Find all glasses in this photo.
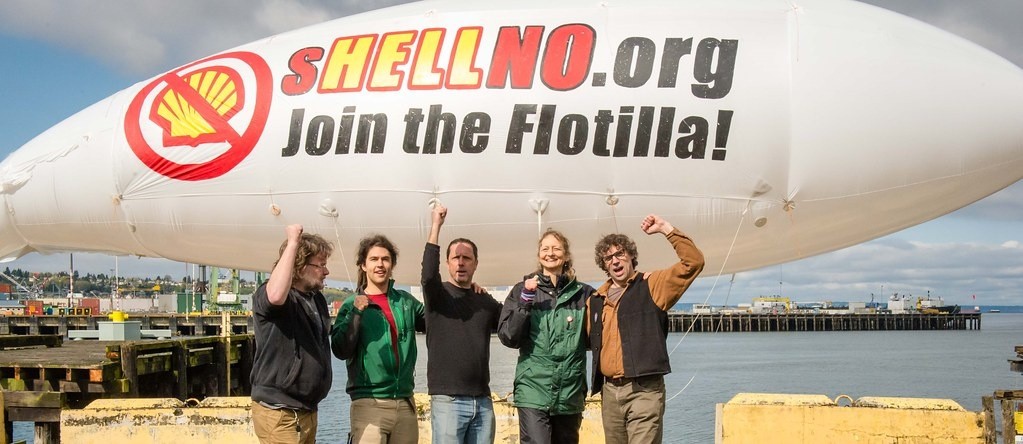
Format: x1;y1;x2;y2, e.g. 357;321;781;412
306;262;326;271
602;247;626;264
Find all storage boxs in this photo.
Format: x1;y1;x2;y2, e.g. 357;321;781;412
22;300;44;314
81;299;101;316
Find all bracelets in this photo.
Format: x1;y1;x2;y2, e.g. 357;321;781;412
521;293;535;301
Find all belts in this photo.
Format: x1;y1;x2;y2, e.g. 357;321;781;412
607;375;633;386
404;397;415;412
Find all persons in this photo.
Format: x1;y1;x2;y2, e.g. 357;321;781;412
331;234;426;444
249;224;334;444
585;214;705;444
421;206;504;444
497;230;597;444
